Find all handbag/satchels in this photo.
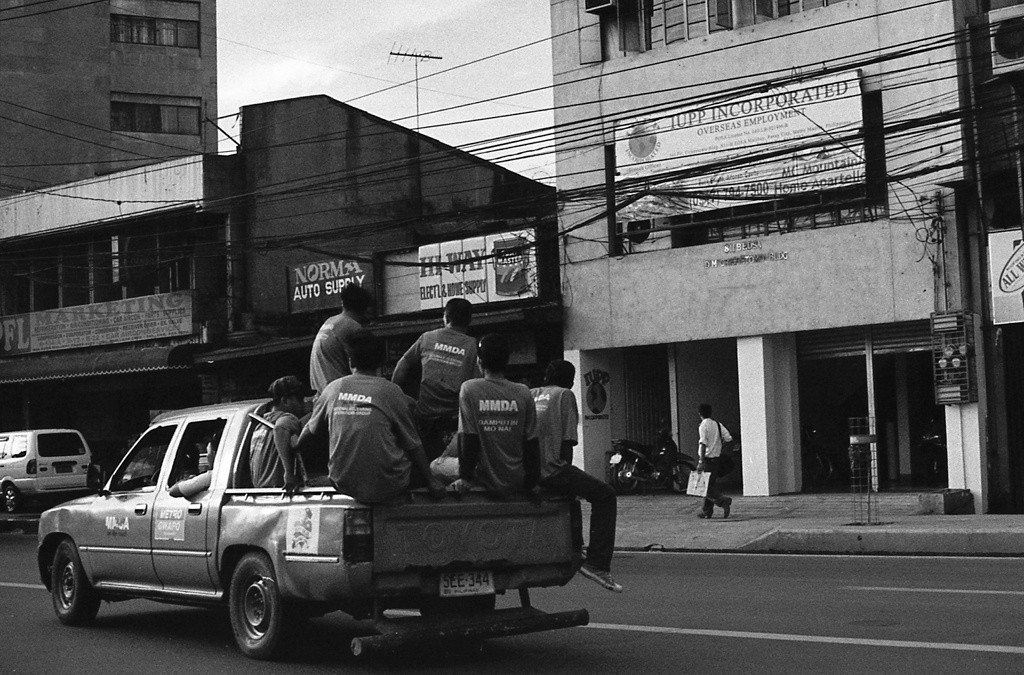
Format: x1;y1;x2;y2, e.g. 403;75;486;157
686;470;713;498
715;454;734;478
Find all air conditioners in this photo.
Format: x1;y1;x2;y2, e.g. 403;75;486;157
622;218;672;254
988;4;1024;75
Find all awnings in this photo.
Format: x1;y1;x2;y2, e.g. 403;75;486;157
0;343;207;385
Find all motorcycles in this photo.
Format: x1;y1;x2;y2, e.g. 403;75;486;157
607;420;696;495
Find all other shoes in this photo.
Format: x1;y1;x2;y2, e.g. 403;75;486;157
723;498;733;520
697;511;709;519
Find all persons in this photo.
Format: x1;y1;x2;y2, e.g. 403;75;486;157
693;402;733;518
290;328;447;507
428;332;625;594
249;375;308;497
169;438;218;499
393;296;480;437
310;282;370;394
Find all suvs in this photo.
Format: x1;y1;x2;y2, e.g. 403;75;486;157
0;430;101;514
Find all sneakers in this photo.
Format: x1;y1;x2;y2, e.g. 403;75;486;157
581;563;623;593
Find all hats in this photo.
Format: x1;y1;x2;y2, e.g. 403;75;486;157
269;377;317;400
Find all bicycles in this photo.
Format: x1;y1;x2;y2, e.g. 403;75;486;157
800;428;835;487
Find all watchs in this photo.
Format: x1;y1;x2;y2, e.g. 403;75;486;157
698;459;704;464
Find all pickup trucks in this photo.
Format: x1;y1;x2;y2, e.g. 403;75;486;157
34;396;591;658
